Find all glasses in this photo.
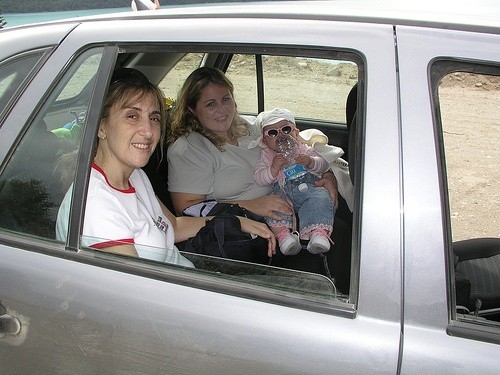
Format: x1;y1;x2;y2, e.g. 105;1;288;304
262;125;293;138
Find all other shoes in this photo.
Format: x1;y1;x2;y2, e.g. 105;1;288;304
307;229;335;255
279;230;302;255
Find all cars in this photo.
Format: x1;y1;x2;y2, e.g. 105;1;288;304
1;0;498;375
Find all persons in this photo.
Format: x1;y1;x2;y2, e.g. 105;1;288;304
165;66;339;257
54;67;278;269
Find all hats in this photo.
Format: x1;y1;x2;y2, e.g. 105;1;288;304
247;108;295;151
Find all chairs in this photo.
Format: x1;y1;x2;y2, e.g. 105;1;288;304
347;81;500;322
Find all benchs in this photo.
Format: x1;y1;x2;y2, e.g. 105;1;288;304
0;109;177;238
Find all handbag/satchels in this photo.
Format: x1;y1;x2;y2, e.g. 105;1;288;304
185;214;272;265
